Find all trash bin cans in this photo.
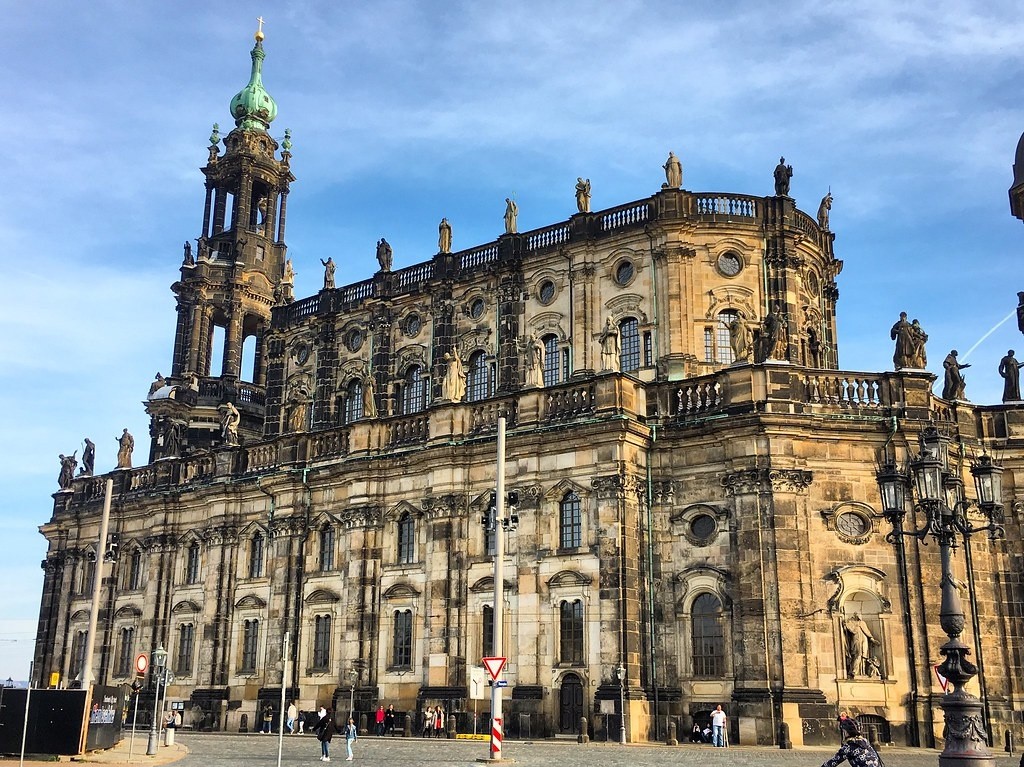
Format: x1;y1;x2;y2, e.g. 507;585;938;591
164;728;175;747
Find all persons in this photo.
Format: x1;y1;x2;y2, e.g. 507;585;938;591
840;610;882;676
148;372;180;457
165;710;181;731
821;718;886;767
438;218;452;254
57;437;96;490
260;700;357;761
773;155;834;231
286;345;466;432
575;177;591;212
891;312;1024;402
375;704;396;737
320;257;337;289
422;706;444;738
662;151;682;188
692;705;726;748
183;234;248;265
721;305;788;364
286;253;294;284
598;316;622;372
221;402;240;446
375;238;392;271
115;428;134;469
516;334;545;387
503;198;518;234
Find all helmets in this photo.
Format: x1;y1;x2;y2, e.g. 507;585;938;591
841;718;861;736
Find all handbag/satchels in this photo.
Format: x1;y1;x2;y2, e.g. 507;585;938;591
317;733;323;741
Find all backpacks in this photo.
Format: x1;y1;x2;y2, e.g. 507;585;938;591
267;708;273;717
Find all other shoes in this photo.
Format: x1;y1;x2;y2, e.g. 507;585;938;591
291;729;294;735
260;730;264;734
319;755;329;761
346;757;353;761
268;731;271;733
297;732;304;734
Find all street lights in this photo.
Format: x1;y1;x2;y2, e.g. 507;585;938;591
475;492;518;764
79;532;119;690
348;669;360;718
146;642;168;754
871;413;1005;767
616;661;627;743
